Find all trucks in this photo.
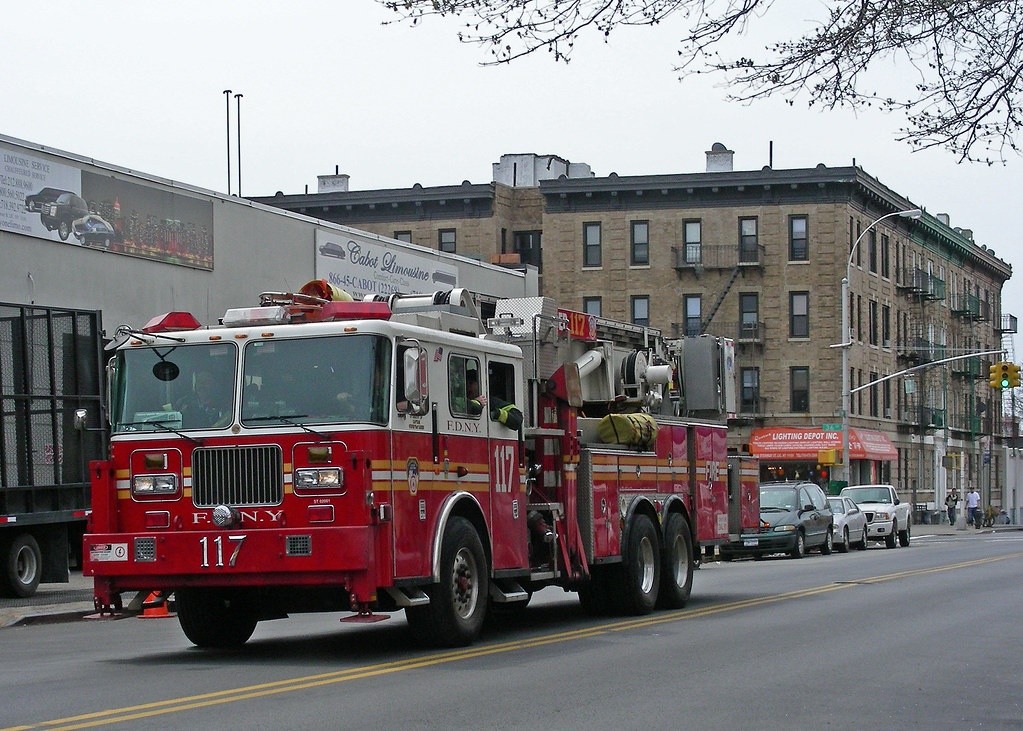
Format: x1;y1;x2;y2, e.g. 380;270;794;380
0;302;109;599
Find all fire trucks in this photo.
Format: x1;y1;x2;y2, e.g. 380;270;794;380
73;276;761;647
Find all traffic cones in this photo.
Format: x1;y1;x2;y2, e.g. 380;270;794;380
135;590;178;619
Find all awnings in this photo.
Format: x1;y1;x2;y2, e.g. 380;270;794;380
749;427;899;461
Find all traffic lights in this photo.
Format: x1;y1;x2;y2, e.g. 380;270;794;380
997;363;1013;388
989;362;998;389
1011;364;1022;387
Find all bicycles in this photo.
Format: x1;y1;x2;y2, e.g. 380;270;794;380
981;506;998;528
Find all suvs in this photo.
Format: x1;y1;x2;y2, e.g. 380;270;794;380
714;478;834;563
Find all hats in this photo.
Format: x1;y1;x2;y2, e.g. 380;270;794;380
466;368;477;382
970;486;974;490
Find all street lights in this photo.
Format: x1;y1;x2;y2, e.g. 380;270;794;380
223;90;233;195
840;206;924;487
233;93;244;197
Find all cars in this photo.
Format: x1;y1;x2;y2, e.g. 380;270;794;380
71;214;115;247
431;270;456;288
39;193;90;241
826;496;869;553
25;187;78;213
319;241;346;260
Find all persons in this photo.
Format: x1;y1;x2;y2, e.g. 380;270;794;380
965;486;981;526
334;356;421;419
945;488;958;525
450;367;523;430
171;366;232;428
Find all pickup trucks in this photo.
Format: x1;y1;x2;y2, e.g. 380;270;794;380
839;484;912;550
